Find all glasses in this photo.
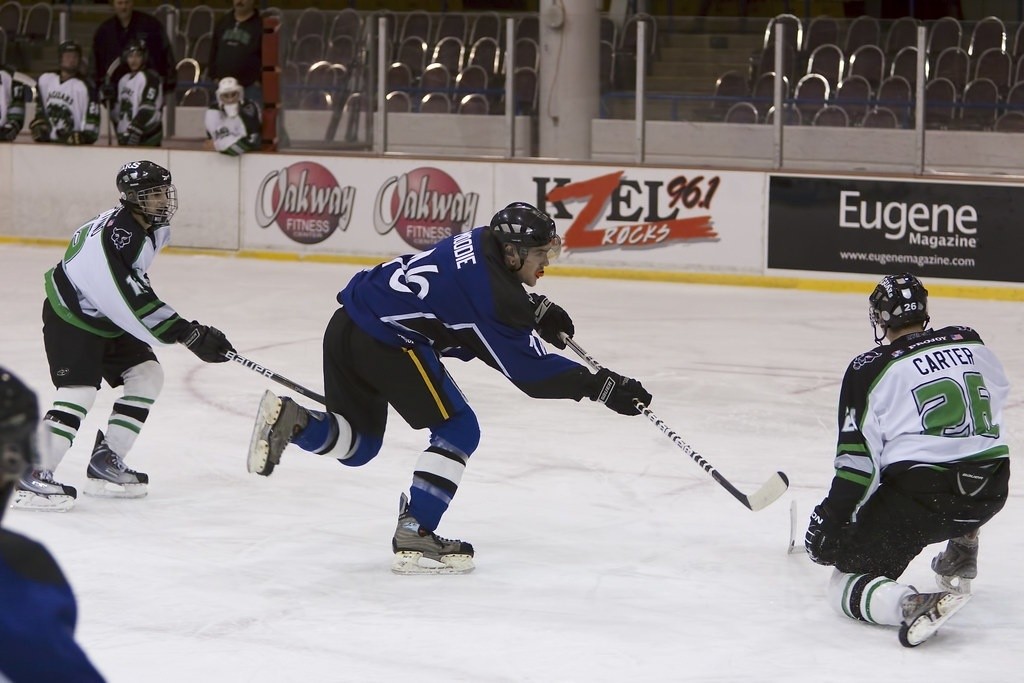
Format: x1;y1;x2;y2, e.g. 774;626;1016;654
220;91;237;99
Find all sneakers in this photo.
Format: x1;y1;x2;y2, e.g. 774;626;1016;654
83;429;149;499
10;468;78;513
248;387;324;477
897;584;974;649
390;493;476;575
930;535;980;596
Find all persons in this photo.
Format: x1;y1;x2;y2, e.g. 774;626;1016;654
9;161;236;511
247;201;653;576
0;367;108;683
1;0;289;157
804;272;1012;650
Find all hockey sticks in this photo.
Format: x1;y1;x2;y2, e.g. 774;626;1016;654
784;500;808;556
560;331;791;512
220;348;328;406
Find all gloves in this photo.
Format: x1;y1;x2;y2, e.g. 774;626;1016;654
530;290;575;351
178;319;237;364
0;121;24;142
118;121;146;146
591;365;653;417
802;496;848;565
29;118;50;142
65;131;96;144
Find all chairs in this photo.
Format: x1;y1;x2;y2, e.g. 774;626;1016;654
710;14;1024;135
0;0;53;68
151;4;658;117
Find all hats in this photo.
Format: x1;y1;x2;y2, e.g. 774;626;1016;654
214;77;245;106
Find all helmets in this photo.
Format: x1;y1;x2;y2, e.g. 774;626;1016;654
868;274;930;329
57;42;81;73
491;201;556;262
116;160;179;227
121;41;149;71
0;367;39;494
